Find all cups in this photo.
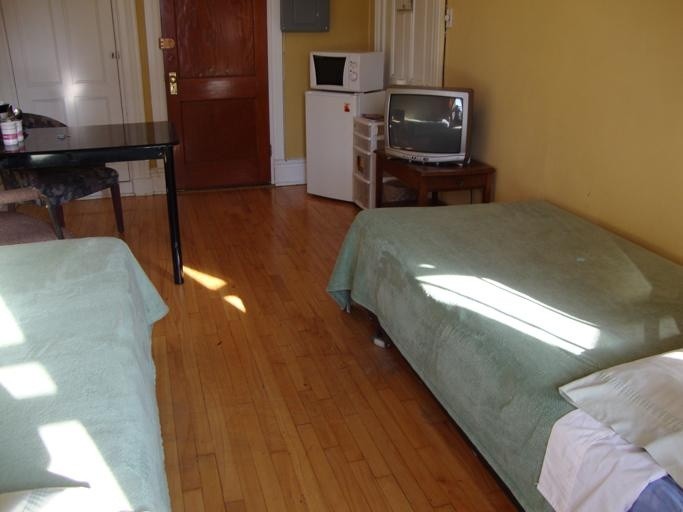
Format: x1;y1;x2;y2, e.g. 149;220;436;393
15;120;25;142
0;122;17;147
4;141;25;151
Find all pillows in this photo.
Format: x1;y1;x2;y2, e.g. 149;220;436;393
557;345;683;489
0;485;93;512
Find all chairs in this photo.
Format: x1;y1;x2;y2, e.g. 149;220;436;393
0;113;125;241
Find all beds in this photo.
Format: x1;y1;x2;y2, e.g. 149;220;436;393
0;238;169;511
323;195;683;511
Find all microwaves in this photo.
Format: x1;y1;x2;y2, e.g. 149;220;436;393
309;51;386;94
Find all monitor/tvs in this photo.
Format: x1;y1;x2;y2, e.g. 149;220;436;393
384;86;474;166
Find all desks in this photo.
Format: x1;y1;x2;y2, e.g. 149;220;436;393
373;148;496;208
0;120;187;284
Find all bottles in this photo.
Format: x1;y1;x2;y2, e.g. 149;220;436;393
0;101;10;121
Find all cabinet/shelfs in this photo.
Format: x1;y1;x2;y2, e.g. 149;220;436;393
353;117;422;210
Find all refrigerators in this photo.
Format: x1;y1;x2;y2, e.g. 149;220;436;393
304;86;390;204
